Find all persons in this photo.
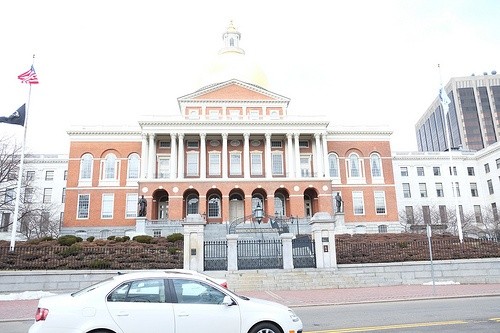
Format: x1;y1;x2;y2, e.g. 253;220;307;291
334;192;344;213
137;195;147;217
174;282;183;302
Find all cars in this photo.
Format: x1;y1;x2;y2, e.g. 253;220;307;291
29;271;304;333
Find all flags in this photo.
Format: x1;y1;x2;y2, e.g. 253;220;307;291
438;82;451;116
1;103;26;127
17;65;42;84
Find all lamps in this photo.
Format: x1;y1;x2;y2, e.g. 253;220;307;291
254;202;264;225
